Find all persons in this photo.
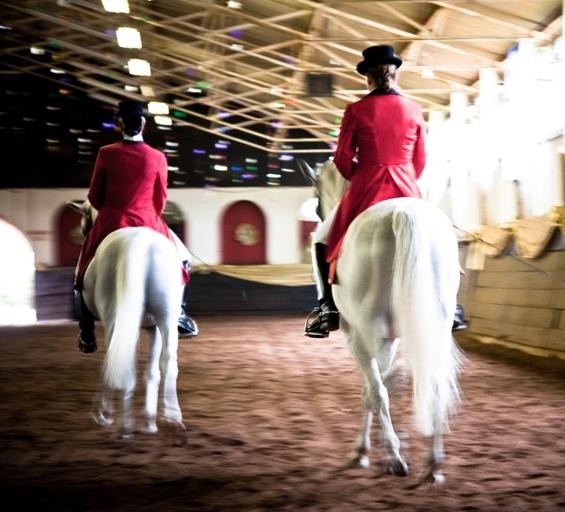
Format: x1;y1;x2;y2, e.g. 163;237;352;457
301;44;473;333
70;95;199;355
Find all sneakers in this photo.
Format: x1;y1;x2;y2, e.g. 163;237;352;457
178;315;200;336
452;305;471;332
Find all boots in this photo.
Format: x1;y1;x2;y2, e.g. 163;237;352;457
74;328;100;355
304;300;342;335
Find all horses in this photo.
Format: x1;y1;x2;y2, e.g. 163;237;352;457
66;197;190;441
297;155;471;487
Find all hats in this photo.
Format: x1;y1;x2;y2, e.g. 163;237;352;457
112;99;151;119
354;43;403;75
